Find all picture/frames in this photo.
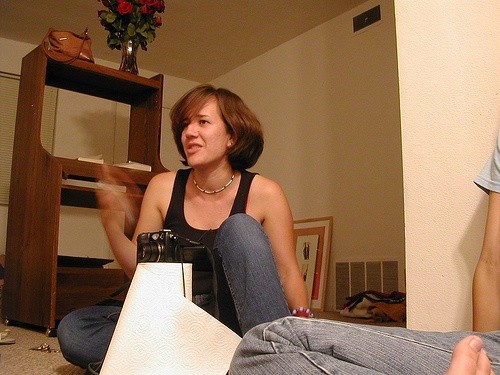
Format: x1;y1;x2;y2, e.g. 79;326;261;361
292;216;333;312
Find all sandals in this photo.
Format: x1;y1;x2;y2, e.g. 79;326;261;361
0;329;16;344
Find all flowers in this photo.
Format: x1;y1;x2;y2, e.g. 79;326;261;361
97;0;167;51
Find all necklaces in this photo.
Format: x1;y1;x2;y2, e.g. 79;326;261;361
192;172;234;194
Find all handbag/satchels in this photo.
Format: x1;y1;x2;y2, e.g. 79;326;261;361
341;290;406;318
40;26;94;65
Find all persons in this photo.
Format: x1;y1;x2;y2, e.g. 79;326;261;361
447;336;495;375
227;134;500;375
57;84;314;368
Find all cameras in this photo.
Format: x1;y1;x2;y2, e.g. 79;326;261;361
136;231;180;265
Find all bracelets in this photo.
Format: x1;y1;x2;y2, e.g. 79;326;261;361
291;308;313;318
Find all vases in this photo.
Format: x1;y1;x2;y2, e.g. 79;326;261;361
118;41;141;74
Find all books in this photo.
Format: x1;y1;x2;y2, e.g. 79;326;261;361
115;162;151;172
77;153;104;164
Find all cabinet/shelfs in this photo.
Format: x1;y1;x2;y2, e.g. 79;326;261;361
3;45;169;330
